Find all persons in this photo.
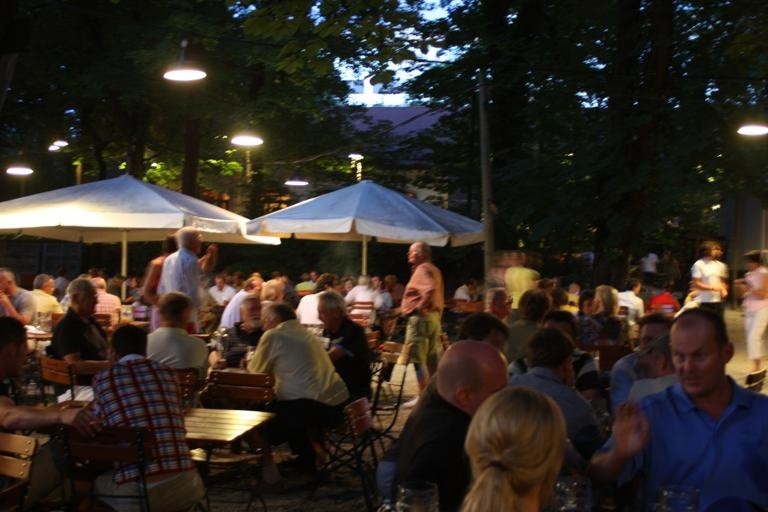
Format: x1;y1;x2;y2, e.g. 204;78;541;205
144;227;217;333
1;267;209;511
374;240;768;512
207;269;404;480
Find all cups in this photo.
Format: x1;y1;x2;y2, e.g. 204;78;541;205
659;486;699;512
553;476;592;511
378;479;440;511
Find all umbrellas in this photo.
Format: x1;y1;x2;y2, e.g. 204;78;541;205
0;173;282;302
245;179;486;276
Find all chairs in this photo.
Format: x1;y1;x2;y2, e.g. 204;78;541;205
0;284;642;512
